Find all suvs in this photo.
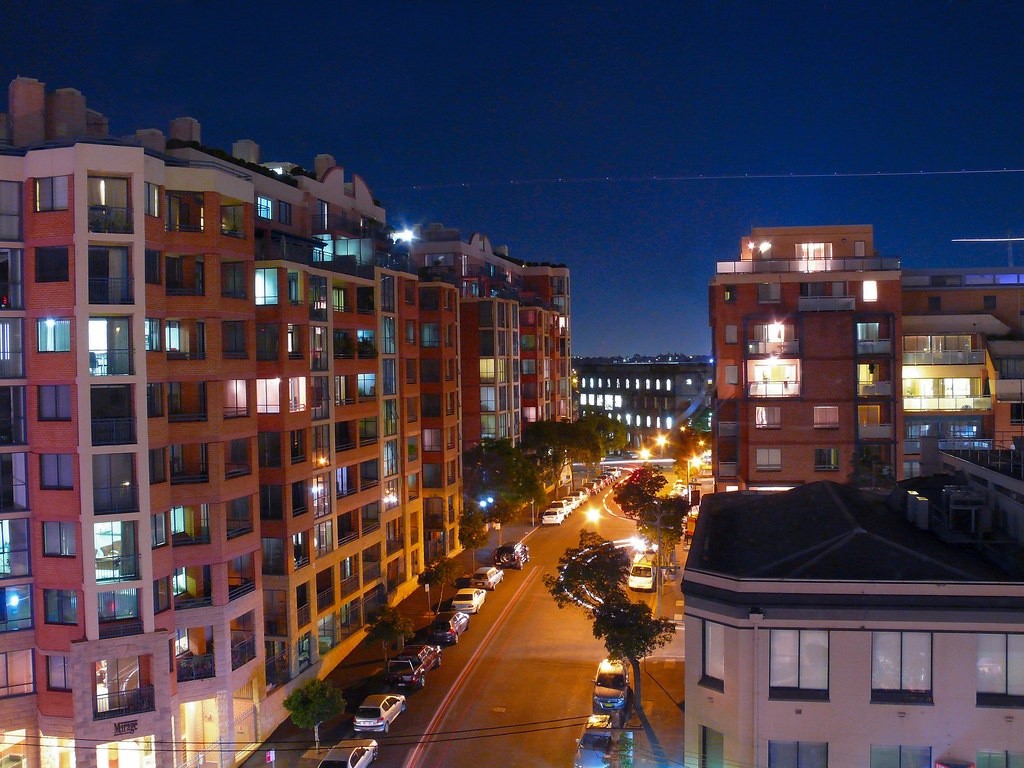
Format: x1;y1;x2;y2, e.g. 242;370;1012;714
591;658;631;713
551;500;572;518
572;715;615;768
494;541;531;570
430;610;470;644
387;643;443;690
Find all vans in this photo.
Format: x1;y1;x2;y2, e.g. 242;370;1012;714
628;553;658;593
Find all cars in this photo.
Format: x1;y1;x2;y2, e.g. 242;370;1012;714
542;509;566;526
471;566;505;592
623;450;638;459
562;467;622;509
317;739;380;767
451;588;487;614
353;694;406;735
